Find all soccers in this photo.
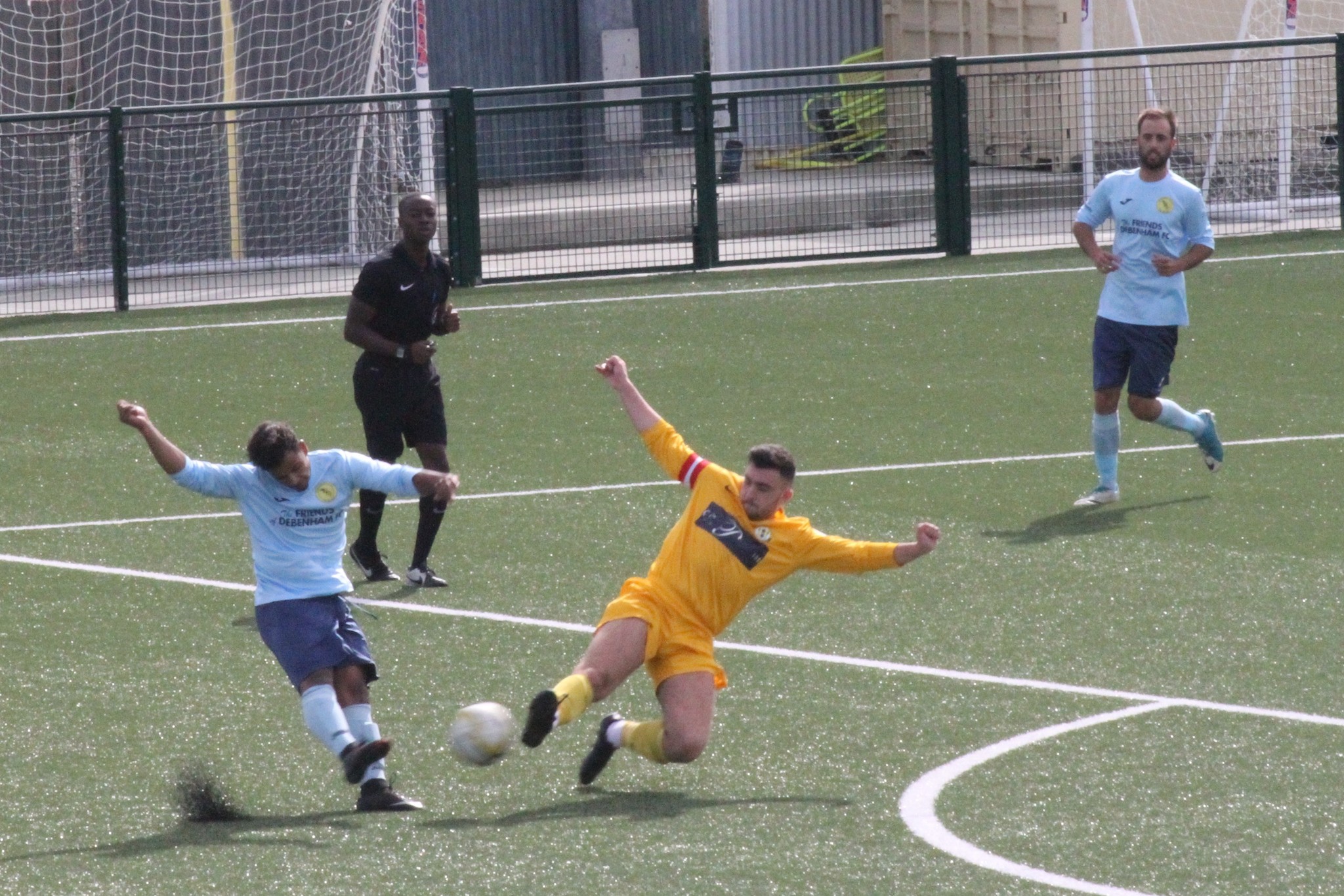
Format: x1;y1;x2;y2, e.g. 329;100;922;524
445;700;515;768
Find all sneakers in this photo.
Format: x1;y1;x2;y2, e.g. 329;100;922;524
349;540;400;581
579;713;623;784
522;690;557;747
405;566;448;587
1194;409;1223;472
1074;484;1121;507
339;738;391;783
357;789;422;810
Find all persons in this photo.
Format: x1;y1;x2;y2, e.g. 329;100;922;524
344;192;460;588
518;353;941;785
116;400;459;812
1072;108;1225;507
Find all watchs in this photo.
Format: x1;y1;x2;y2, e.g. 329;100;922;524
396;344;406;360
